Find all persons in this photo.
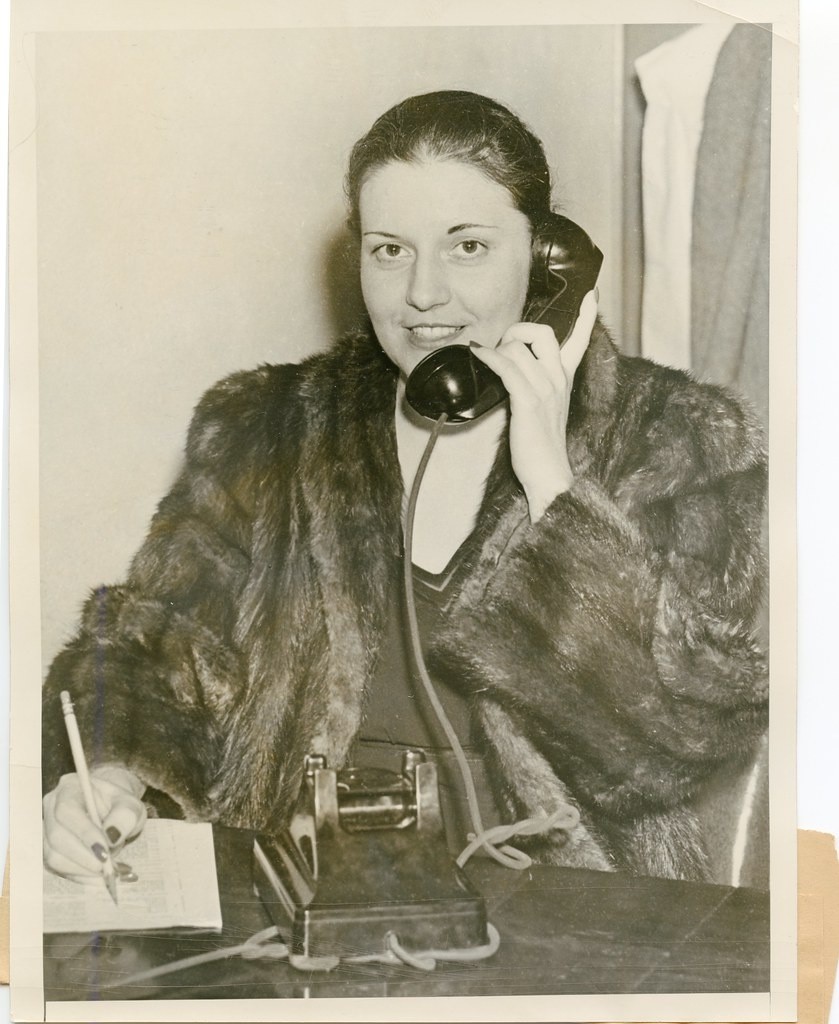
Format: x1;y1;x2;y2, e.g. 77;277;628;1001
42;89;769;881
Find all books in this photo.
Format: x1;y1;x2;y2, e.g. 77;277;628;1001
43;819;222;936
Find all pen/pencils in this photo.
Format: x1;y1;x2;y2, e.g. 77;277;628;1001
60;690;119;906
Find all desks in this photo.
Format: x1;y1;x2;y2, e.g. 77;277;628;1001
44;823;771;1001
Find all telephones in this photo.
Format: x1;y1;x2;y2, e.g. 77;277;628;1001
403;212;605;426
